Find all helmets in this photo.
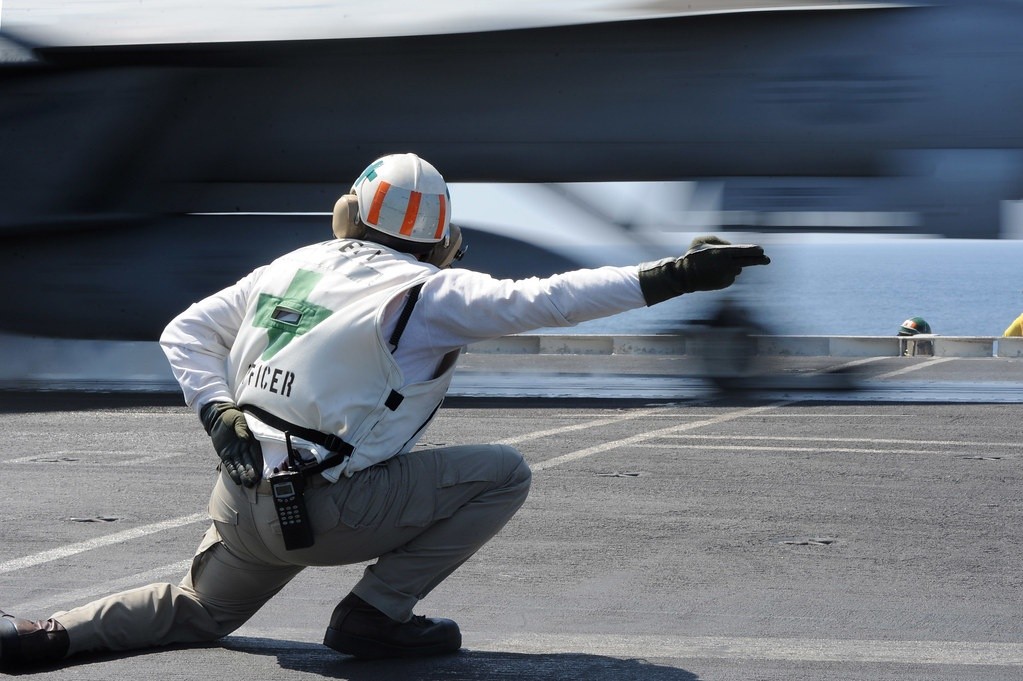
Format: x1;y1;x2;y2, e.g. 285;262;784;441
896;317;932;336
346;152;451;242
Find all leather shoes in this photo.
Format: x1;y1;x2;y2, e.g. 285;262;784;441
0;607;70;675
323;591;462;657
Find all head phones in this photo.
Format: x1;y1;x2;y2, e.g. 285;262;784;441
331;194;469;269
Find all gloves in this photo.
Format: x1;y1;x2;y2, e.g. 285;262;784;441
203;403;260;488
638;235;772;308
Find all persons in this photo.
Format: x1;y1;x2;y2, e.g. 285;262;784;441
0;153;771;675
897;317;931;356
